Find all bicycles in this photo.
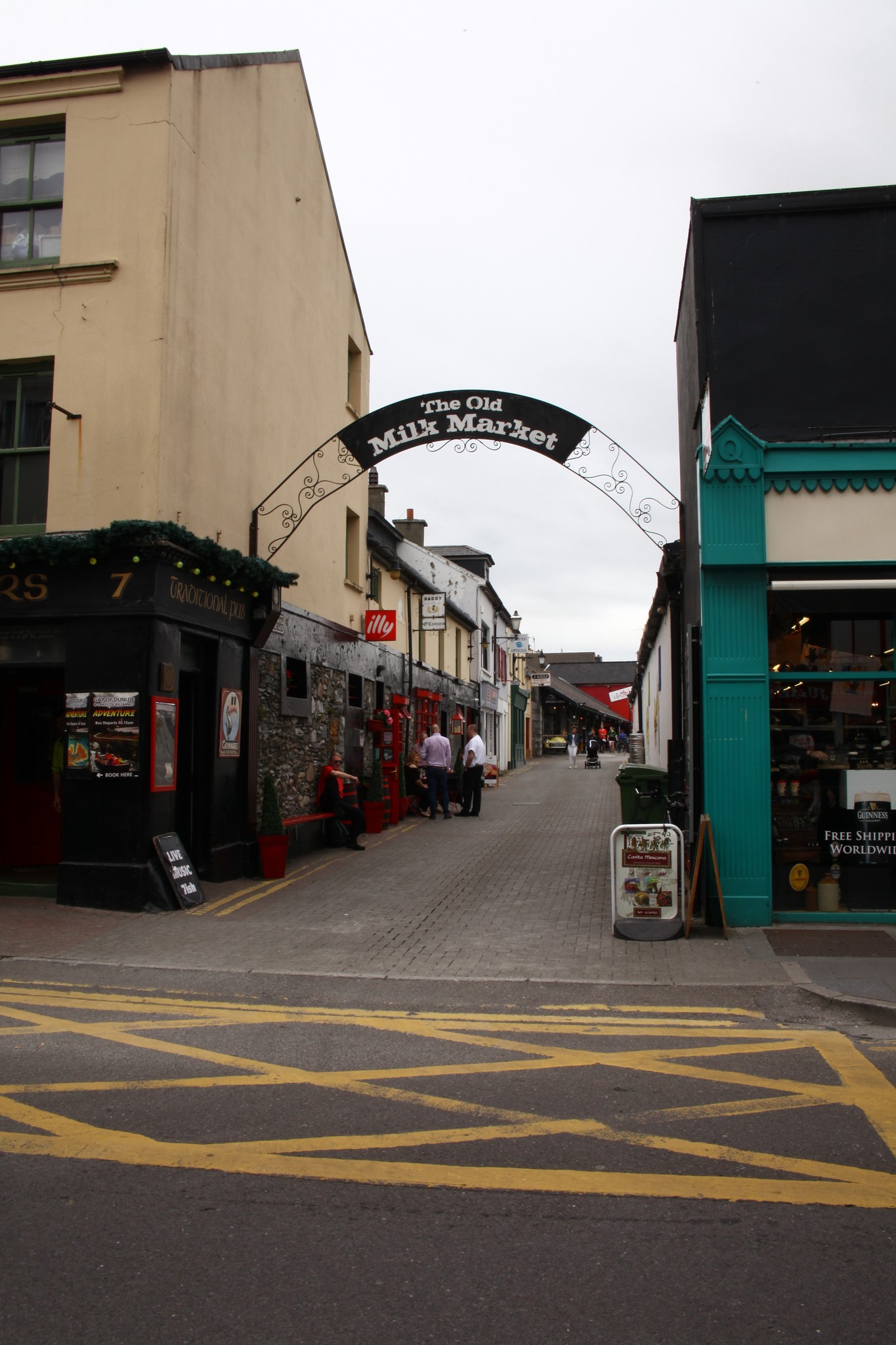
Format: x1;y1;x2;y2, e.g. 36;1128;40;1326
616;737;630;754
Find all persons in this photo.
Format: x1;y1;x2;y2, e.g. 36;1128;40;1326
485;763;497;776
598;725;607;753
484;763;494;776
566;725;579;769
404;750;431;817
588;727;598;753
618;730;628;752
413;732;430;767
453;724;486;817
607;727;618;753
420;724;453;820
317;751;366;851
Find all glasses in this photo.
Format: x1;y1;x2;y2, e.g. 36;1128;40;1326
329;760;343;764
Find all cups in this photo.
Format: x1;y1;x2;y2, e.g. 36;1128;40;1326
777;781;786;796
853;792;891;863
790;781;799;795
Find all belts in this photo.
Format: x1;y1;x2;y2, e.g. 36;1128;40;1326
426;766;446;769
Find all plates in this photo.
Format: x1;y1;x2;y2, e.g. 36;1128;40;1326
625;881;639;891
634;892;649;906
657;891;672;906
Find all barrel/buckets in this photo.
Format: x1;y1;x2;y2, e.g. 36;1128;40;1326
628;734;645;764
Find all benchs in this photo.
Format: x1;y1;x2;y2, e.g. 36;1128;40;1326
282;812;335;841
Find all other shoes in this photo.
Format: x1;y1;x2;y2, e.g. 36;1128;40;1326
612;751;614;753
569;765;573;769
600;749;604;753
429;812;436;820
420;811;431;817
468;811;479;816
346;839;366;851
444;814;452;819
332;804;345;816
454;809;469;817
609;750;611;753
573;763;577;768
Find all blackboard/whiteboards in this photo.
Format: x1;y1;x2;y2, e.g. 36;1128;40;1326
155;832;207;909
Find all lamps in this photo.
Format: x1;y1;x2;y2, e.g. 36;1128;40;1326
468;628;490;663
366;545;403;601
512;649;546;681
492;610;522;652
451;713;464;734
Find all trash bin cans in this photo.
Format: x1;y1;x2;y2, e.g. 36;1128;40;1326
615;763;671;825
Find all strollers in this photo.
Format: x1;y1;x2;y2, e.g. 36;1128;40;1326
585;740;601;769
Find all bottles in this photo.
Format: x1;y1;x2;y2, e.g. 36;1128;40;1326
807;837;818;848
806;883;818;911
819;873;839;912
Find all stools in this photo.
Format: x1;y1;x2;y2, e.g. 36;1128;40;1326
409;791;459;817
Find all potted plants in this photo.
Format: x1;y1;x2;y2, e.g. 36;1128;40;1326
398;752;409;821
258;774;289;878
363;759;385;834
